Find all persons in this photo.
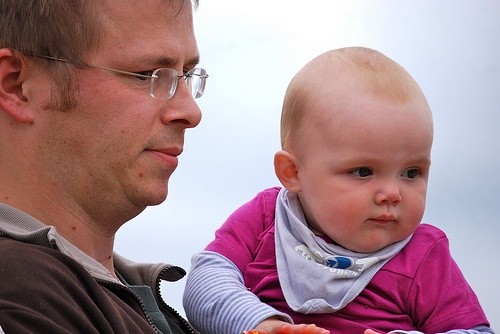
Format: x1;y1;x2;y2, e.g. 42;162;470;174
183;47;492;333
1;0;208;334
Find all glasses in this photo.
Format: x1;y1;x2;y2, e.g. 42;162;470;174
19;51;208;101
